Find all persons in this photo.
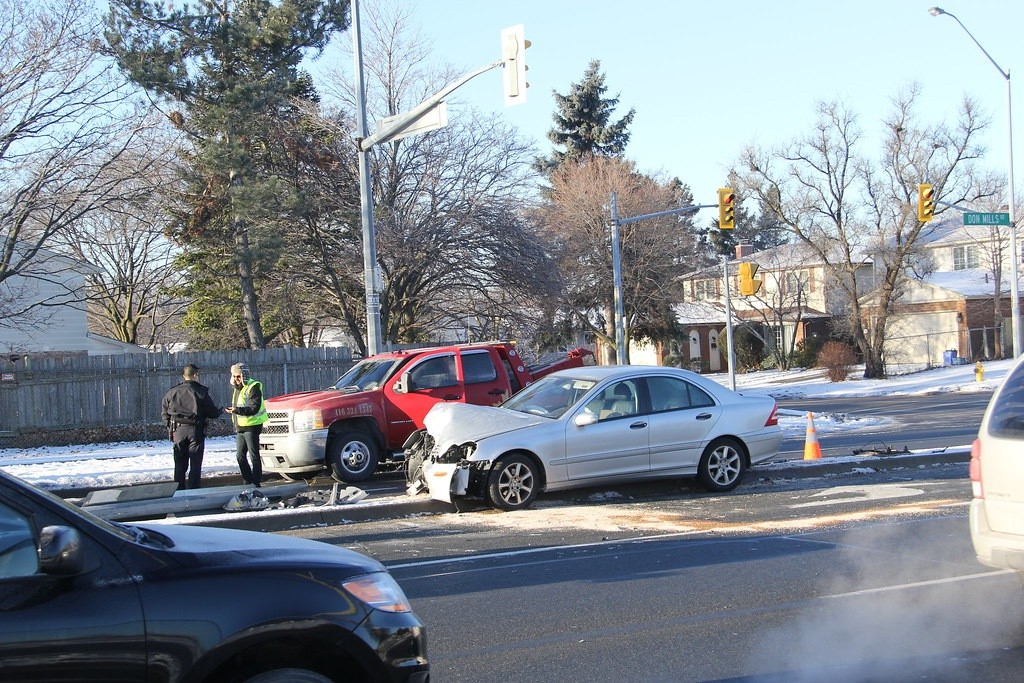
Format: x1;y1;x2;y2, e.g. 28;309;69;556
225;362;268;487
162;364;225;490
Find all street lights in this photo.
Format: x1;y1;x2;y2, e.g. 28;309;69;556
929;8;1023;359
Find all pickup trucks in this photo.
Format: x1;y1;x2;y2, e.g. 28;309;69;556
250;340;601;484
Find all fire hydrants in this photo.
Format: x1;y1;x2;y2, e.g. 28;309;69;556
974;361;984;382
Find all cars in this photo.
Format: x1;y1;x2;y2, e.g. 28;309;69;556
413;365;782;516
1;470;433;683
967;356;1024;572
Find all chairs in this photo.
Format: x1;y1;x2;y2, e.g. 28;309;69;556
587;389;605;416
610;382;636;414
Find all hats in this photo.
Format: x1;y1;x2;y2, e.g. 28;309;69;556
230;363;250;386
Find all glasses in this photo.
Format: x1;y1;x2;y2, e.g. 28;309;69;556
233;375;240;378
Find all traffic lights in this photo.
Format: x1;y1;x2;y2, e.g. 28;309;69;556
719;188;737;230
501;21;534;100
739;261;768;300
916;183;936;221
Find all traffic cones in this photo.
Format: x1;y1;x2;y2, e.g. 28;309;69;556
803;410;827;462
